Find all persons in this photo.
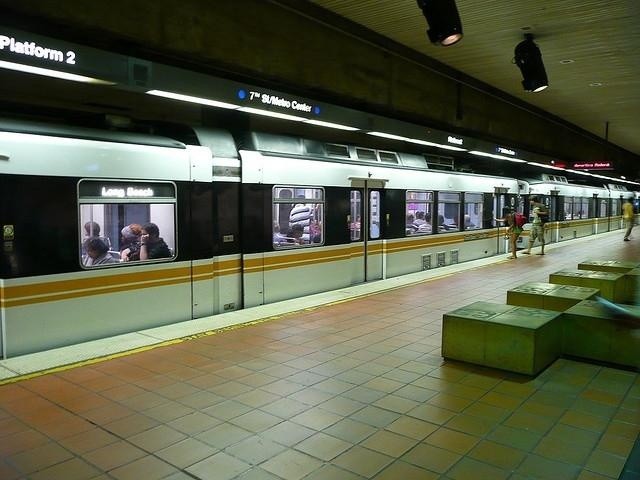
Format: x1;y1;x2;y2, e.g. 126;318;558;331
521;195;548;255
406;210;476;236
81;221;172;266
272;194;313;247
566;211;574;218
494;206;524;259
621;197;634;241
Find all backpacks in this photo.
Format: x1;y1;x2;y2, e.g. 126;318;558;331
512;212;527;228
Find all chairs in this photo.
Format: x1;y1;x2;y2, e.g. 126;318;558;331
81;236;174;264
275;236;298;248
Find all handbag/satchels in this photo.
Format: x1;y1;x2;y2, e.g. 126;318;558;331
539;207;550;223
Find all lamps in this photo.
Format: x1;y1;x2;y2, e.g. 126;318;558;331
417;0;549;93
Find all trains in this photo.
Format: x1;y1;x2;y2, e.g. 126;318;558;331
0;118;639;364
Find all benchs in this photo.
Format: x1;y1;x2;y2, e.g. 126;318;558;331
441;259;640;375
